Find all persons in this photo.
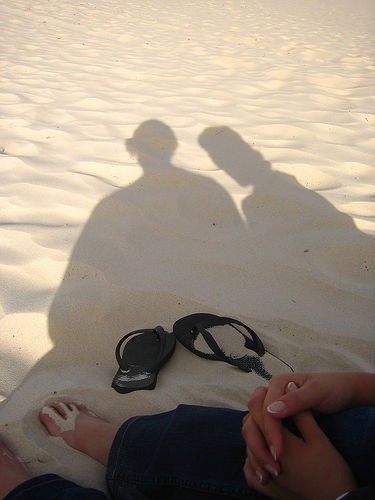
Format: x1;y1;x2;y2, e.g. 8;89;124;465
0;372;375;500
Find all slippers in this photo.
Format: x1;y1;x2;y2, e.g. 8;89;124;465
173;313;295;383
110;325;175;394
